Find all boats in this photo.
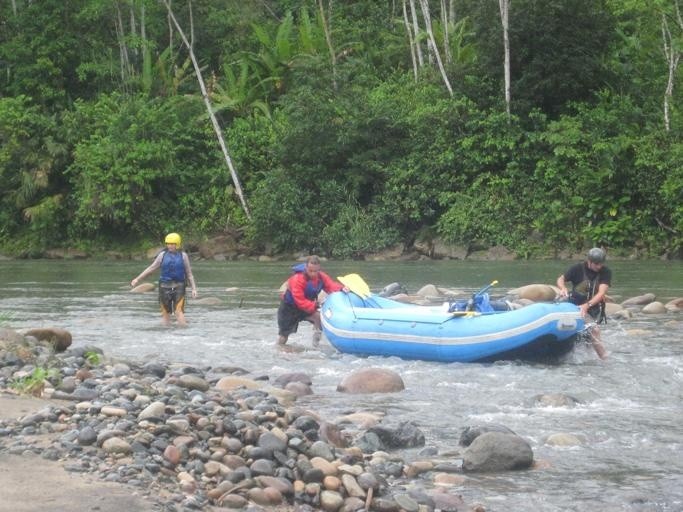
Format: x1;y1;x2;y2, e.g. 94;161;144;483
319;290;585;363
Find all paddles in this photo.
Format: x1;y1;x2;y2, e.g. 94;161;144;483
337;273;381;307
453;311;507;317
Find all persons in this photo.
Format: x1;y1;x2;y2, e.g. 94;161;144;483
276;255;350;348
557;247;613;361
131;233;197;327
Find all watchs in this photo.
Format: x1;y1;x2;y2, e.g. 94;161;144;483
587;302;592;308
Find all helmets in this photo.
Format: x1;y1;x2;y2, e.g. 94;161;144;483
589;248;606;264
165;233;182;250
